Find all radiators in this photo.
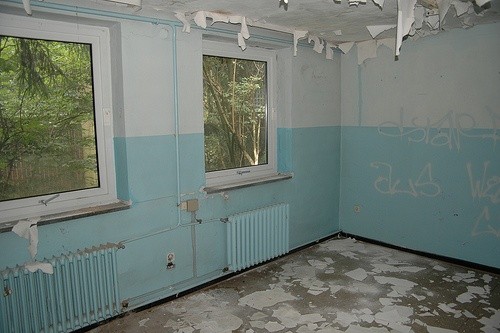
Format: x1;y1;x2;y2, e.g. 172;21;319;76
226;200;290;272
0;242;128;333
